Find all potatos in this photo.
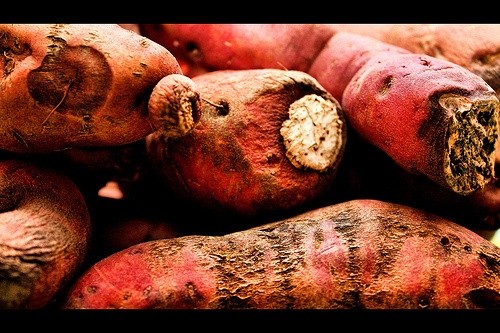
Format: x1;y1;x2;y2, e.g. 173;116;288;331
0;24;500;310
